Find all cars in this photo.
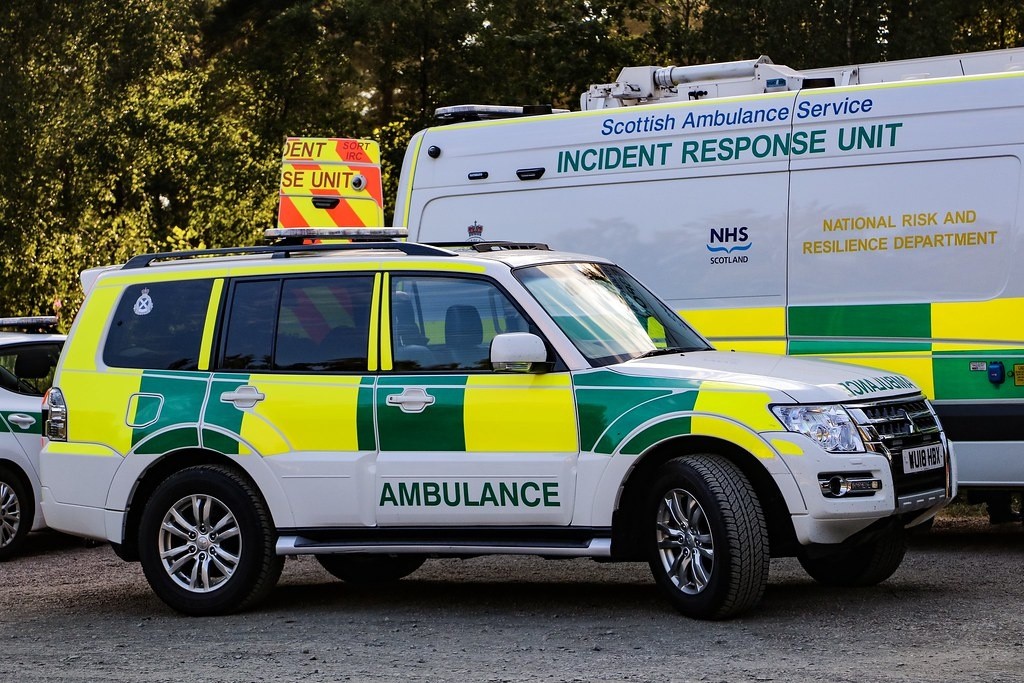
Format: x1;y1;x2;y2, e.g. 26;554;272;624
0;315;78;563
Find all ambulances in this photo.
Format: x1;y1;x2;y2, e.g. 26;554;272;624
394;43;1024;536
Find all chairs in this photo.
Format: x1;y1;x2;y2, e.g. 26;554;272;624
444;305;490;370
393;291;441;367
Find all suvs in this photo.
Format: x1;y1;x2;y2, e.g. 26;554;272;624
37;227;961;621
